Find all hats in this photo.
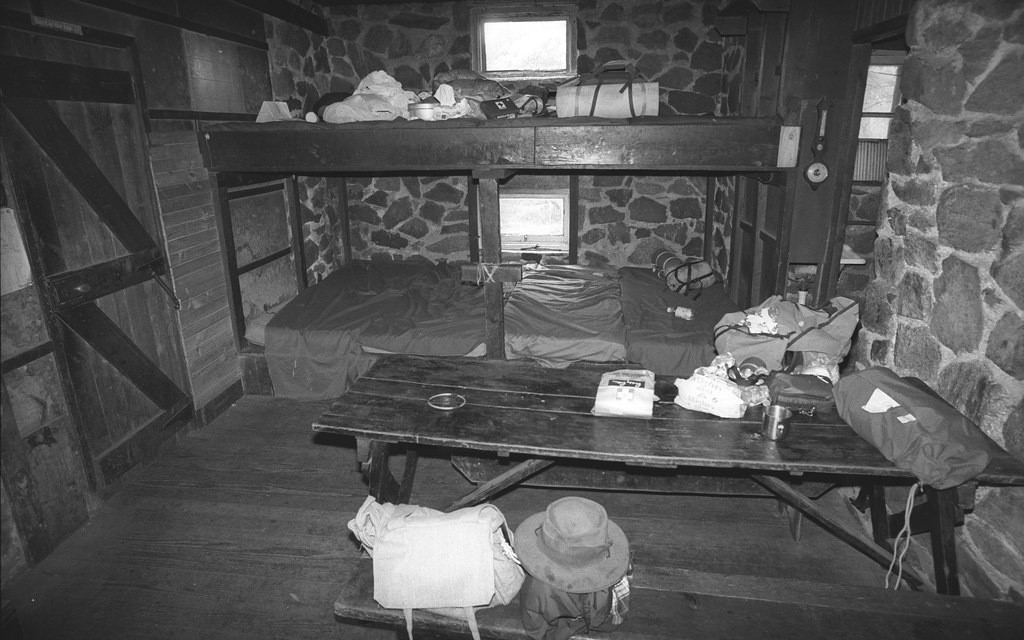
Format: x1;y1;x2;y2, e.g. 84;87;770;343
513;496;629;593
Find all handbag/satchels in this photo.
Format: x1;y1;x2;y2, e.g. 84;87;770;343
765;370;835;416
479;97;518;120
665;261;715;300
347;494;526;640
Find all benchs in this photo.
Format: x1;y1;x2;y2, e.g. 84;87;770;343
332;451;1024;640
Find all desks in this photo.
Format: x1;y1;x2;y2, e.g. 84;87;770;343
311;353;1024;596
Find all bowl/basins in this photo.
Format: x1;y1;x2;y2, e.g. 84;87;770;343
409;103;442;121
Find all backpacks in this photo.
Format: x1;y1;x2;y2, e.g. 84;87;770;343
714;294;859;372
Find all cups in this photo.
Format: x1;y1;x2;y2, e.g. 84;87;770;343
762;406;793;442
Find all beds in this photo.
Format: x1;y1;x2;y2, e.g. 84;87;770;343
197;115;796;399
